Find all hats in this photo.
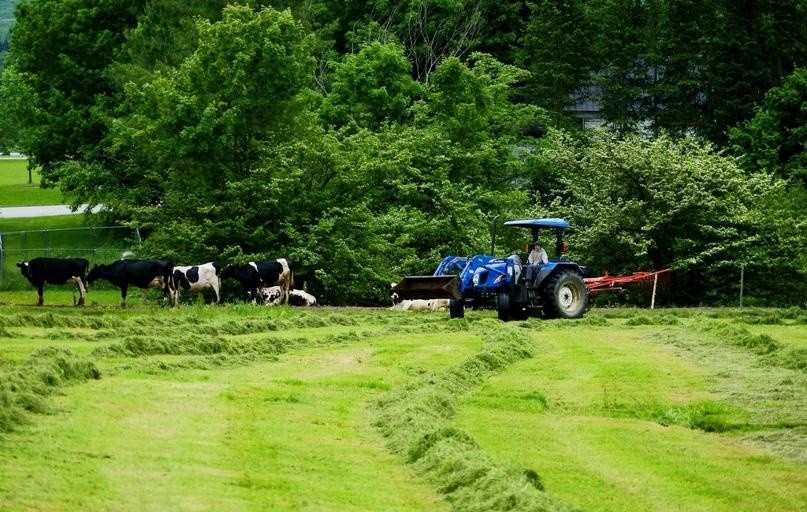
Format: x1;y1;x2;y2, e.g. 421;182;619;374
535;241;542;245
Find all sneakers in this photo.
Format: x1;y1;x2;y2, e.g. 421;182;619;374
525;280;532;286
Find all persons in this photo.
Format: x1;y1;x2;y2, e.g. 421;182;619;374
524;240;549;286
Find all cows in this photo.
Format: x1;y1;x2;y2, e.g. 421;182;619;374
86;258;176;308
219;257;295;304
385;282;451;313
247;285;317;307
16;256;89;307
162;262;222;309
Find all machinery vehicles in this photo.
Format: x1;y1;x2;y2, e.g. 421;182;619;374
388;217;674;318
